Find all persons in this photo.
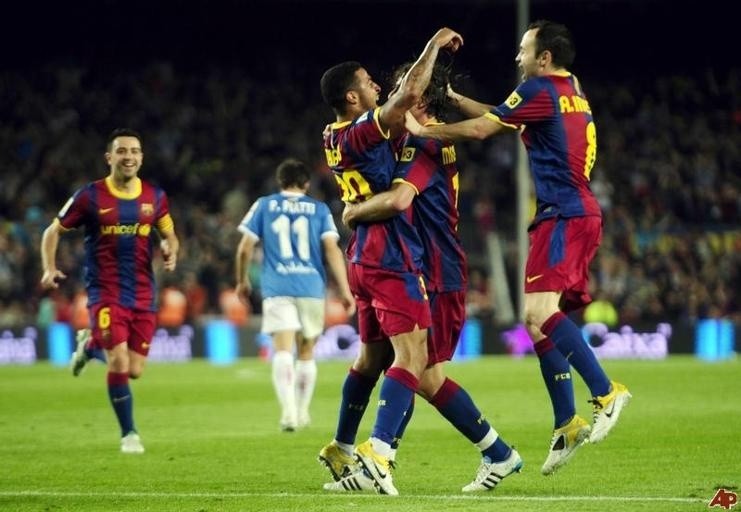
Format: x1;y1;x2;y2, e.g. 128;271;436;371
234;158;357;432
39;128;178;455
319;27;463;496
404;19;633;477
322;54;522;497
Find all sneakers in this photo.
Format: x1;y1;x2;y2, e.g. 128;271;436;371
460;446;526;493
316;440;358;479
352;439;399;496
69;328;92;380
119;432;145;456
589;378;632;446
321;465;383;494
541;413;592;477
281;414;298;434
